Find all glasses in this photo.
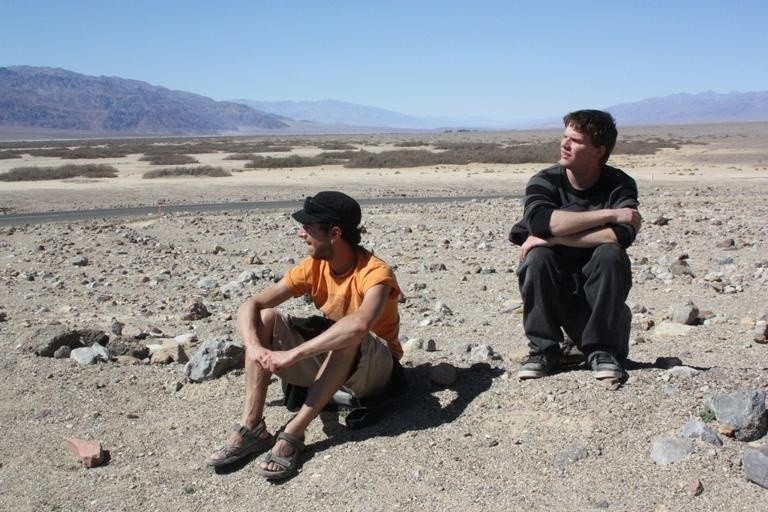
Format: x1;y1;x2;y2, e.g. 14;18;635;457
304;196;331;214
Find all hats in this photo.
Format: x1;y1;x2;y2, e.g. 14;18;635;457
291;191;361;226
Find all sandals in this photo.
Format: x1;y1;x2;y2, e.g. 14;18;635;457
258;430;304;479
205;418;274;467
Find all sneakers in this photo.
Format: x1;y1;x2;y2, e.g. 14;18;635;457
585;350;622;378
517;356;561;378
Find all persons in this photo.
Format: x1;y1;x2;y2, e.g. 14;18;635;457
206;190;401;479
508;110;643;380
